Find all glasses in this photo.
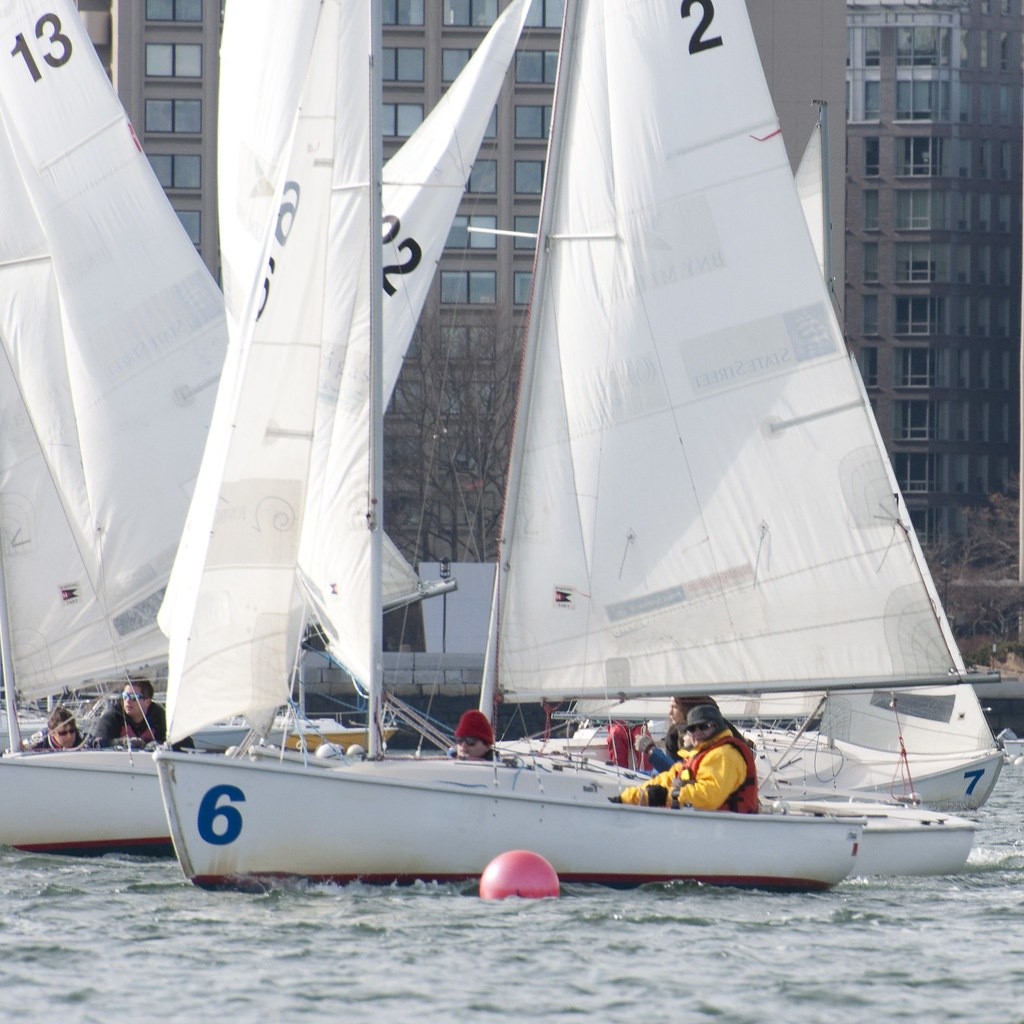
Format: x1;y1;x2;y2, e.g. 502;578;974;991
52;728;76;736
456;737;477;746
687;722;716;734
122;691;143;701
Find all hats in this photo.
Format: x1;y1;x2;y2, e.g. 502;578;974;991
454;710;493;744
684;705;722;727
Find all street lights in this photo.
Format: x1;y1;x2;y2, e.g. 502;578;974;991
439;556;451;653
941;559;953;617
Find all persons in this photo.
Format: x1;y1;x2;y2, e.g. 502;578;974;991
448;710;499;762
608;720;653;776
94;677;195;754
32;706;104;751
607;705;759;814
634;696;757;773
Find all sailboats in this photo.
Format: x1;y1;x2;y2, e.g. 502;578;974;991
0;0;1007;893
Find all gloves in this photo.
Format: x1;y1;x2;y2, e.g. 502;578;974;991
634;726;656;755
